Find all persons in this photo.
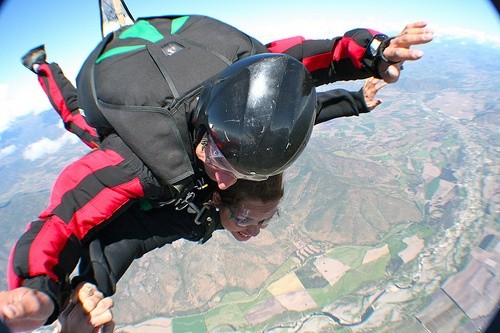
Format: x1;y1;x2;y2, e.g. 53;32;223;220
56;78;391;333
0;15;433;333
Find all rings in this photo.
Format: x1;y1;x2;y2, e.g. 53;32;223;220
87;287;93;296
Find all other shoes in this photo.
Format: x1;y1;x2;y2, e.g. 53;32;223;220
21;44;48;74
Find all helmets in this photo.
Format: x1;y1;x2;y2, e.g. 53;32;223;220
191;52;317;176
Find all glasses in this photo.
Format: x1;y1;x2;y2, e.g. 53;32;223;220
206;129;269;182
227;205;285;226
